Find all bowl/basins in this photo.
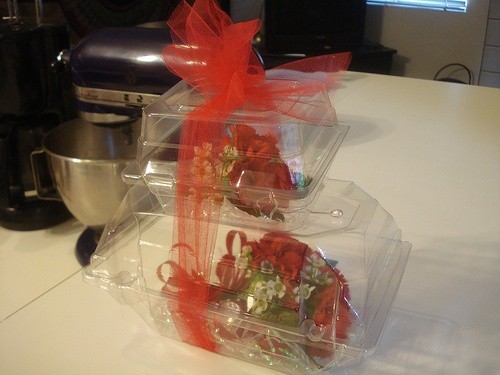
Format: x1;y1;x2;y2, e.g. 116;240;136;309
30;117;173;276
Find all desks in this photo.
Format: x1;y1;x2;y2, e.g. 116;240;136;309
0;70;500;375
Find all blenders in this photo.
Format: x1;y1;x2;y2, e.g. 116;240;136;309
50;27;266;143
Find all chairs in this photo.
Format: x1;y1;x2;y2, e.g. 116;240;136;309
251;1;398;78
